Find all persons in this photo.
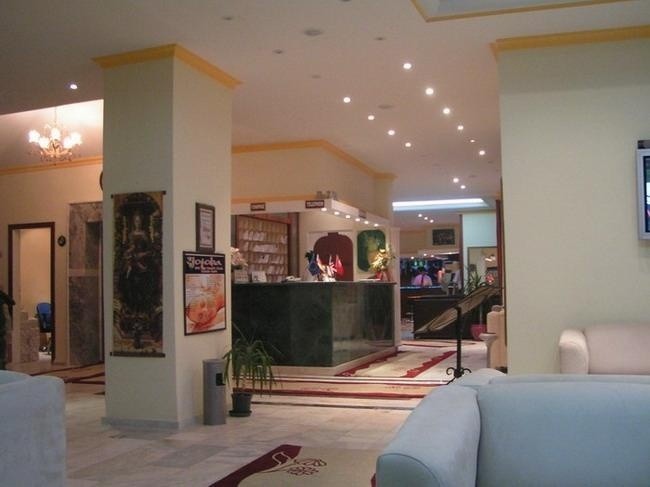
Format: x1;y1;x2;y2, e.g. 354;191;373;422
186;286;224;332
412;267;432;287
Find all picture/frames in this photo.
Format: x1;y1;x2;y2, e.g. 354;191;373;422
181;201;227;335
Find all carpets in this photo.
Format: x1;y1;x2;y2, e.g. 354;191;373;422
335;348;457;378
206;443;377;486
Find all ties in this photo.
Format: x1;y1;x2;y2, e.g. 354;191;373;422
421;274;425;286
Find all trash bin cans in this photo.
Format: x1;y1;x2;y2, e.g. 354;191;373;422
203;358;227;425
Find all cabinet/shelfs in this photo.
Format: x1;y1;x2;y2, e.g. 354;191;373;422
235;213;292;282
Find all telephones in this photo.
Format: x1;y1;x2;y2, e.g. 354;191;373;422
286;275;301;282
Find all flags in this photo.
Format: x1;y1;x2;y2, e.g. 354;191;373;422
306;253;344;277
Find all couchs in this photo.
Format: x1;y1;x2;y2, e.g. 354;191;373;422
558;324;649;376
376;367;649;485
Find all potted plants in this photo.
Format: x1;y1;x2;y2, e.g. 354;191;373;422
221;321;288;416
462;267;494;341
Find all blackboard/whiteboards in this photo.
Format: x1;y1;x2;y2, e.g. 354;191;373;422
432;229;455;245
